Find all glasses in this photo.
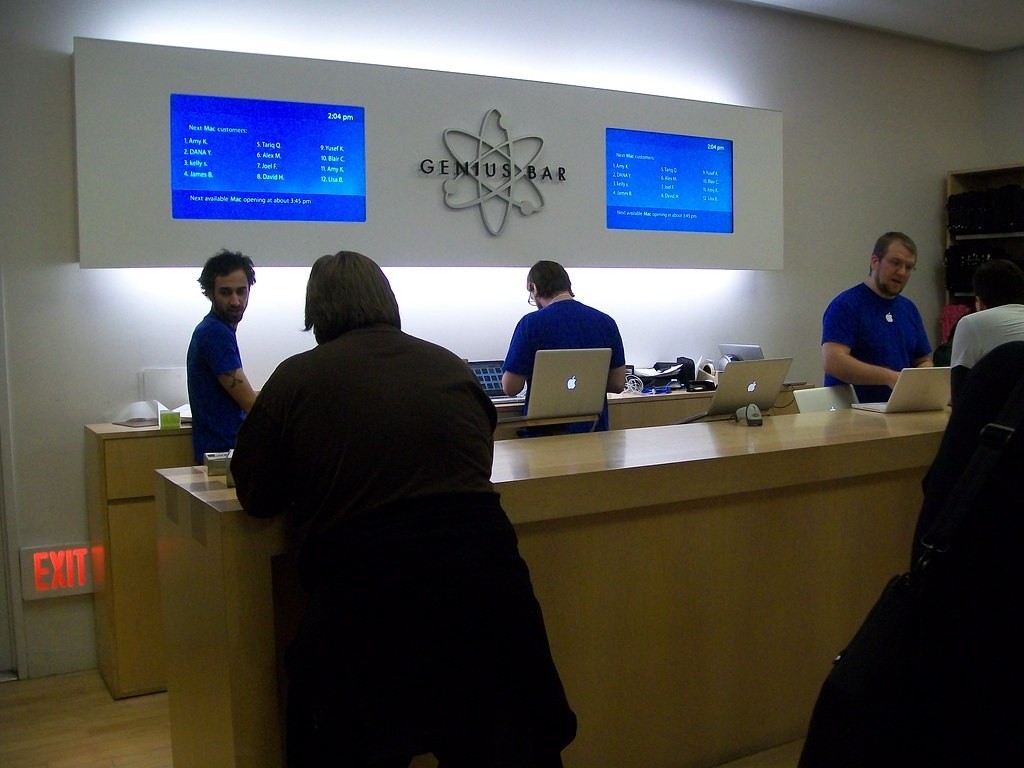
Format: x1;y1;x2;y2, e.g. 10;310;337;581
878;255;916;273
528;292;536;306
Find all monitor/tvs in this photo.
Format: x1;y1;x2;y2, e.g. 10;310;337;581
170;93;366;222
607;128;734;234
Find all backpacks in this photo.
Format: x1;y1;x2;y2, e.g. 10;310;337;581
939;185;1024;342
793;341;1024;768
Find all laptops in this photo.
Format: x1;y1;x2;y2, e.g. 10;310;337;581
678;344;794;426
793;384;859;414
497;347;612;423
851;367;951;413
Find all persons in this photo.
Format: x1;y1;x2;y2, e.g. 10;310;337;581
819;231;933;405
948;260;1024;369
186;252;257;466
228;249;576;768
501;261;627;439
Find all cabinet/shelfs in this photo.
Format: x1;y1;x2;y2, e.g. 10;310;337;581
86;384;817;702
945;163;1024;304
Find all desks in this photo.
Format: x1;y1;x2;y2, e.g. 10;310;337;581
153;405;952;768
